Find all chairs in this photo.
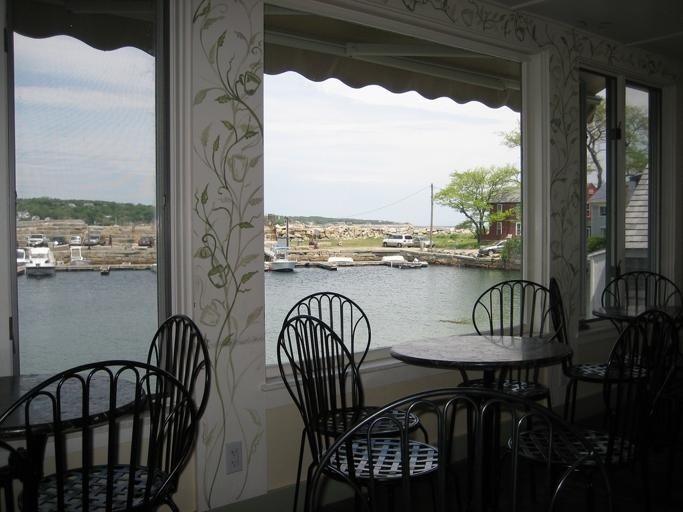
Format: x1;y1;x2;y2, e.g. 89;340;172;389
0;363;200;511
16;313;212;510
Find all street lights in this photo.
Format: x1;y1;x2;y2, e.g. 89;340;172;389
284;216;290;247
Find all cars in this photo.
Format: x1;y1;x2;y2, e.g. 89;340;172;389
413;236;434;247
137;236;154;246
478;240;507;256
51;236;65;246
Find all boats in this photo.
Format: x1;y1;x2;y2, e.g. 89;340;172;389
24;246;57;276
265;243;296;272
16;247;29;275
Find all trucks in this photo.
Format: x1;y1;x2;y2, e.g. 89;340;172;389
84;230;105;246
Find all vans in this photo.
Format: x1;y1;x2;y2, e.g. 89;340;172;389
382;234;414;247
69;235;82;245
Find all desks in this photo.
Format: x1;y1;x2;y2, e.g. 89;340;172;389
0;374;146;512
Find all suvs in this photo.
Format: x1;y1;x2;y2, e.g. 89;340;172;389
26;234;48;247
